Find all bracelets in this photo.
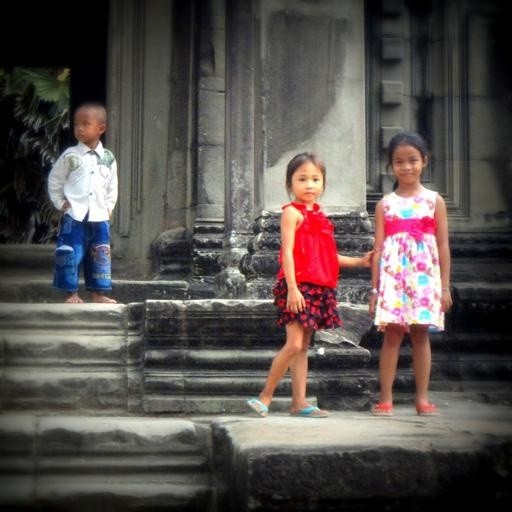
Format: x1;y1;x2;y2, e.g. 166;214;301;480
442;289;449;292
368;288;378;294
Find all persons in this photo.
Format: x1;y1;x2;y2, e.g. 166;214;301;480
368;131;453;416
248;153;378;417
48;104;120;302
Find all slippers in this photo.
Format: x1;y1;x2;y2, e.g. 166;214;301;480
372;403;394;415
290;406;328;417
417;403;438;416
247;398;268;416
